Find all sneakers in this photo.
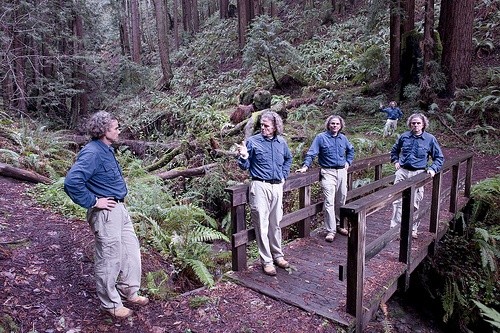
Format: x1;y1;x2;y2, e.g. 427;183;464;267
262;263;276;275
337;227;348;235
325;233;336;241
412;231;418;238
126;291;149;305
274;257;290;268
100;305;133;318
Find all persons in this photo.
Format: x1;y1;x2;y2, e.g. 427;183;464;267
379;100;404;140
390;112;444;242
63;109;150;320
234;110;292;276
294;114;355;243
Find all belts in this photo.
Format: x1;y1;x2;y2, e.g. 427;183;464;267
387;118;396;120
401;165;425;171
322;166;345;169
252;177;281;183
109;197;124;202
